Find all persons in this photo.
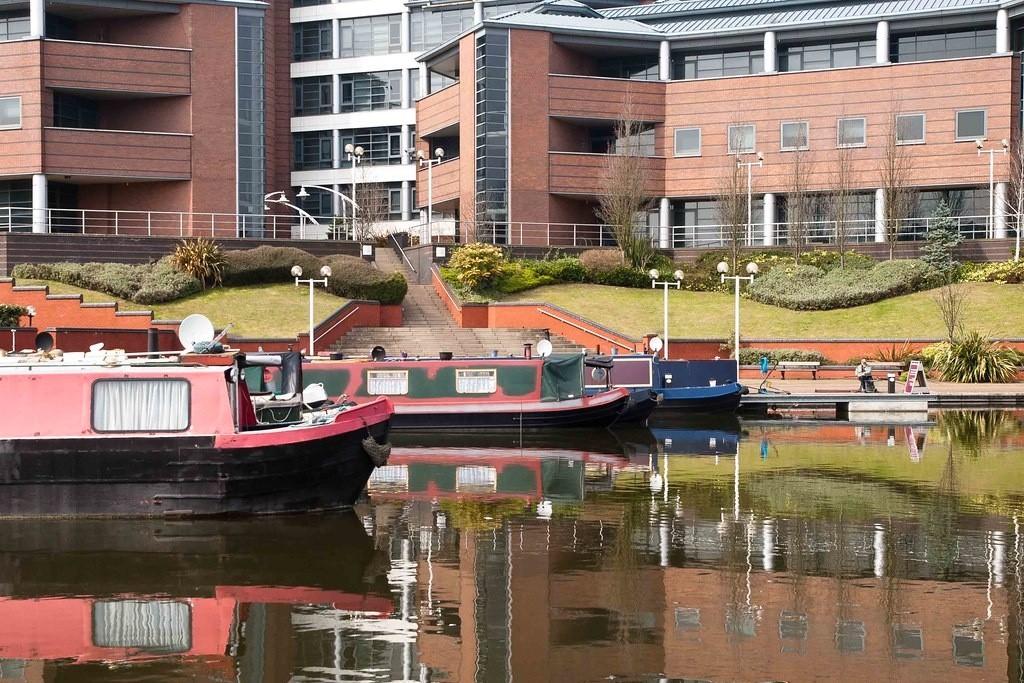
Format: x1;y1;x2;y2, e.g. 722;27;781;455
854;358;877;393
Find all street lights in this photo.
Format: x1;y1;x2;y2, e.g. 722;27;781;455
25;305;37;326
417;148;443;242
648;268;685;359
344;143;364;241
263;200;319;239
291;264;332;357
975;138;1009;238
296;184;363;240
736;151;764;246
718;260;759;384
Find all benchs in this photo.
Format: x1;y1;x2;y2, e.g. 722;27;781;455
776;362;821;380
865;361;906;376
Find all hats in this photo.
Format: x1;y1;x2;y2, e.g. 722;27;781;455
861;358;866;362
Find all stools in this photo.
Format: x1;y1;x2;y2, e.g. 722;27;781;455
858;379;876;393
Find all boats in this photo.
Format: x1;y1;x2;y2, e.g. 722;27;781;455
246;339;630;426
583;356;657;420
584;337;741;412
0;313;396;518
